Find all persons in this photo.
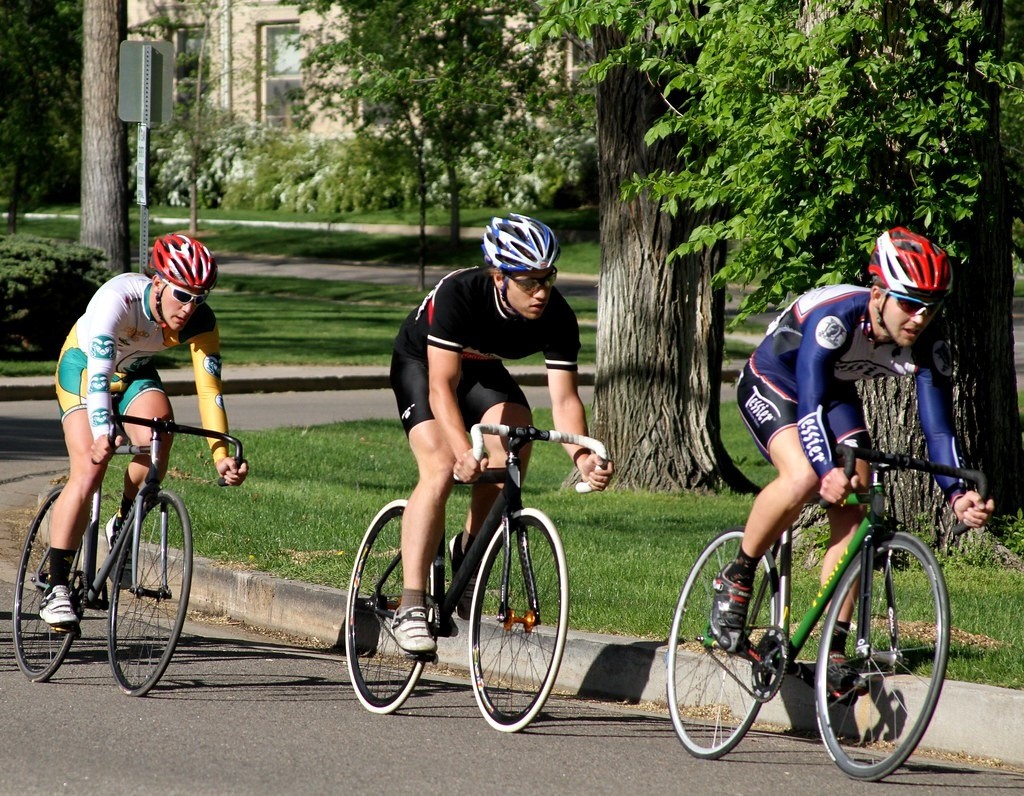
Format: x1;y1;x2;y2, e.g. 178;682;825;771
389;213;615;652
710;228;996;701
38;233;249;628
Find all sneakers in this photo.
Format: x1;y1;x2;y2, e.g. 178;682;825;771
38;585;79;626
105;511;133;589
391;605;437;654
448;532;481;621
825;651;869;695
710;561;753;654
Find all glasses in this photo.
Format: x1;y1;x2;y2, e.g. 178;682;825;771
500;265;557;296
155;270;210;306
880;289;944;317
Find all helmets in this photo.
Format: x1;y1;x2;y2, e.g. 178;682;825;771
869;226;953;303
481;214;561;271
150;232;218;295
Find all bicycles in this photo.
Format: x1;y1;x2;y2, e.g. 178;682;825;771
345;423;610;733
666;439;990;782
12;415;244;698
